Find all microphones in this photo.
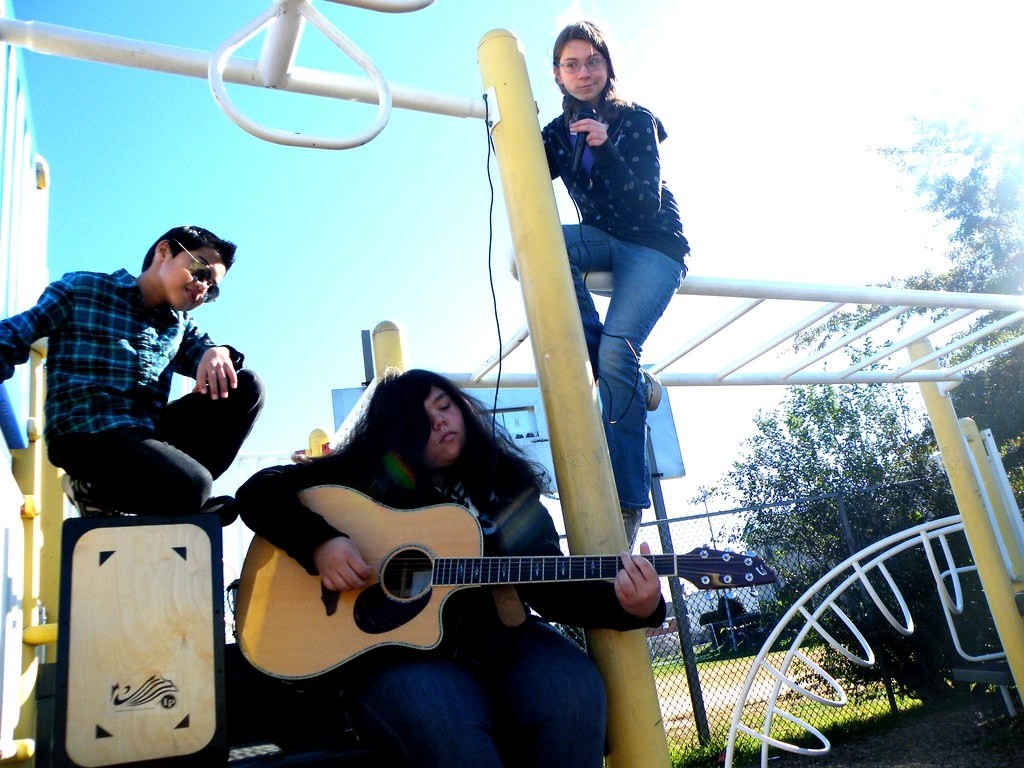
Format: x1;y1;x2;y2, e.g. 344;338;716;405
569;107;597;189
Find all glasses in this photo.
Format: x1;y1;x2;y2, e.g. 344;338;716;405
174;239;220;303
556;58;606;73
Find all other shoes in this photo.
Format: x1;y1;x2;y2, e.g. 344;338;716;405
61;474;116;519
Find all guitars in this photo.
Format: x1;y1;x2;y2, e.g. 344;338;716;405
235;484;777;690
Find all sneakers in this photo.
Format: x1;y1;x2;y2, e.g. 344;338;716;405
640;368;661;411
621;511;642;554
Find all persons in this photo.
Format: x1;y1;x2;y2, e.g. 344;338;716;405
508;16;695;555
233;370;667;768
0;224;261;529
